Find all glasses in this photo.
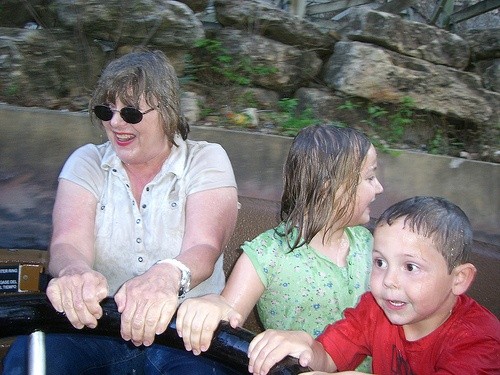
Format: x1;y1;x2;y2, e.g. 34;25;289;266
93;102;165;123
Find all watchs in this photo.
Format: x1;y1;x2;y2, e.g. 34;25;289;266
153;257;191;299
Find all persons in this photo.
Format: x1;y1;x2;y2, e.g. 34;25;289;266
176;123;383;375
3;45;240;375
248;196;499;375
